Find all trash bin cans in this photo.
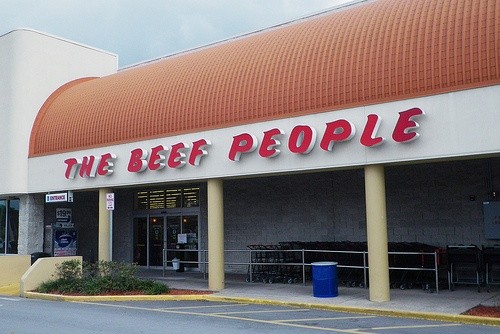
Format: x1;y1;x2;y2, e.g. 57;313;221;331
310;261;340;298
30;252;51;266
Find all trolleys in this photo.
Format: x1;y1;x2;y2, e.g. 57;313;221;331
246;239;500;295
